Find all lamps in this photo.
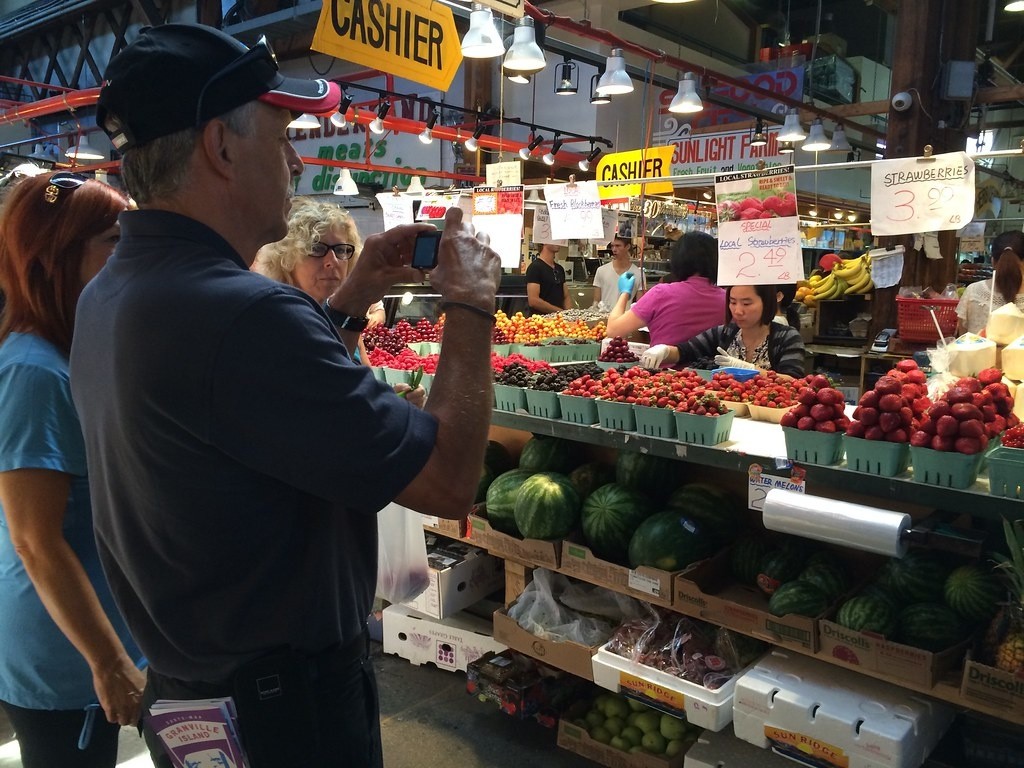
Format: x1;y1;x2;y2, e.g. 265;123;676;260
668;72;703;114
748;103;856;155
286;84;602;197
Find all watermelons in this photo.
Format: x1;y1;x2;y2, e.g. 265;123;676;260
470;432;1001;651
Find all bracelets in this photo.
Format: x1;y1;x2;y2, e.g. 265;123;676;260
367;301;386;329
441;301;497;325
322;299;368;332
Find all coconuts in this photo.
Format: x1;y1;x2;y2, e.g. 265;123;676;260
946;302;1024;422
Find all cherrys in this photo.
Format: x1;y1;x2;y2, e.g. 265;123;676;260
362;309;606;357
599;338;640;362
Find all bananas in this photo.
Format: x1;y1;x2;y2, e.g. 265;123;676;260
810;249;874;301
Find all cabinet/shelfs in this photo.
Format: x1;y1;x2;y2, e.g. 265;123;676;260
353;384;1024;768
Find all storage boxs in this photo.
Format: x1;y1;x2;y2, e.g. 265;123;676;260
803;55;893;123
589;44;634;105
817;292;872;345
459;1;547;84
897;296;959;341
372;341;1023;768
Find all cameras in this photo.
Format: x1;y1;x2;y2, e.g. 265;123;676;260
411;230;443;270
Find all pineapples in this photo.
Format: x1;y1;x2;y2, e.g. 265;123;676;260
982;514;1024;678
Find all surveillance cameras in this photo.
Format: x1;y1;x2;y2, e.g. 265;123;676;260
893;92;912;111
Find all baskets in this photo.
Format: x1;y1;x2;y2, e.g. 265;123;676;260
408;340;600;363
374;366;1024;499
895;295;959;340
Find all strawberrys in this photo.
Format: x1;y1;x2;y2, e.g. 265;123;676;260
717;191;796;223
562;364;807;417
779;358;1020;455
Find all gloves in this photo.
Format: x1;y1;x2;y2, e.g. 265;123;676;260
715;347;754;370
617;272;635;301
641;344;671;369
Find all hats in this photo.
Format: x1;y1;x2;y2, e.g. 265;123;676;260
96;23;342;155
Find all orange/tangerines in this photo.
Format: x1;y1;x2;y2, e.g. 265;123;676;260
794;275;822;307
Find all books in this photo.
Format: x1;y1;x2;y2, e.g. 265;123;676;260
151;695;252;768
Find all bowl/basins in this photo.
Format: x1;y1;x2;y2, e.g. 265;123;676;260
711;366;760;383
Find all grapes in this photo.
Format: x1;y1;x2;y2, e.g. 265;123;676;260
1002;421;1024;449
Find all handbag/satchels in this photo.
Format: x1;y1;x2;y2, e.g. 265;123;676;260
376;504;431;605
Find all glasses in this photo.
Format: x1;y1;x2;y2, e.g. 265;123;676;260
196;34;278;128
306;241;355;260
39;170;89;210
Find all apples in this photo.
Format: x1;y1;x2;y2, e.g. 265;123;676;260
572;691;697;762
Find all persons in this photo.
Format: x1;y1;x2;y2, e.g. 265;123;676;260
606;231;728;345
526;244;571;315
772;284;800;330
593;233;647;310
640;284;807;381
70;23;500;768
263;198;425;410
956;228;1024;334
0;175;135;768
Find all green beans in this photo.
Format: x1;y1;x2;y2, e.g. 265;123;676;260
397;365;424;398
